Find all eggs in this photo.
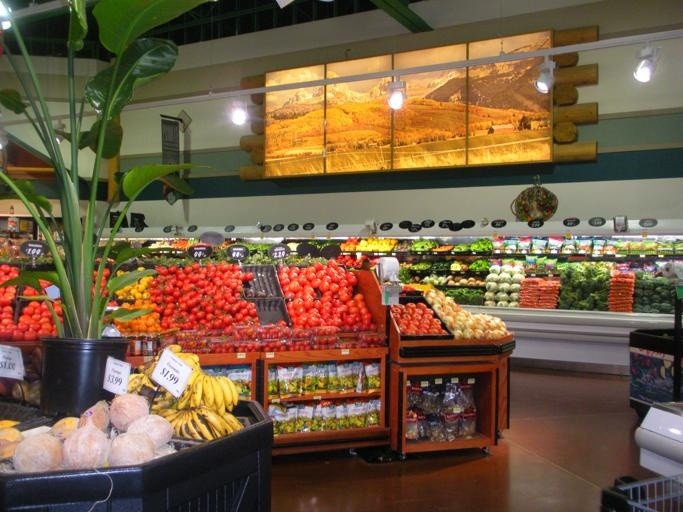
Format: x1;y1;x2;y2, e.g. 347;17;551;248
483;264;525;307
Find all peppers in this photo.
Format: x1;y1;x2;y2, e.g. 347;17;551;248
447;276;485;287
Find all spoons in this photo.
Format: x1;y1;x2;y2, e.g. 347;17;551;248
147;258;387;353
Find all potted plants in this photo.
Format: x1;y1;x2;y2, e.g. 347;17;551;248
2;1;204;420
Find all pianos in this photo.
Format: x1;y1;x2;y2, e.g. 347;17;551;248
519;278;561;309
608;273;636;312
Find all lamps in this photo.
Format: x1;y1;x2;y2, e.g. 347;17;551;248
385;75;406;110
633;41;662;84
1;7;13;30
534;56;556;94
228;100;248;125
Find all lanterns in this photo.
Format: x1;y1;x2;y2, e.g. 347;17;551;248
355;236;399;252
115;266;153;299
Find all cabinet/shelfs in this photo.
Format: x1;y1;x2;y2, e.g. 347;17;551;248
380;219;683;375
605;329;683;511
1;345;274;510
3;213;515;459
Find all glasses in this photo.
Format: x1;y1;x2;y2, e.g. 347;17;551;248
1;7;13;30
385;75;406;110
633;41;662;84
228;100;248;125
534;56;556;94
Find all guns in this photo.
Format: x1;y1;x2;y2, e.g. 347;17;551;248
115;344;246;442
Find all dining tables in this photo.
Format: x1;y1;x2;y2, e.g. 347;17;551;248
2;1;204;420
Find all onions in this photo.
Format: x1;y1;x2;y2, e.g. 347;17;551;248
423;287;508;340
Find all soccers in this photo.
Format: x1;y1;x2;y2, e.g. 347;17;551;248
391;302;448;336
0;263;118;341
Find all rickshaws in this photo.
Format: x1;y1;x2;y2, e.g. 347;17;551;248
1;393;179;473
